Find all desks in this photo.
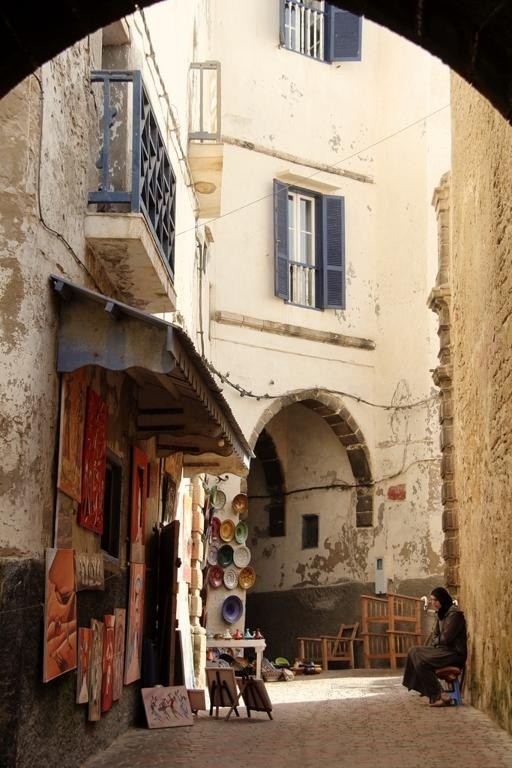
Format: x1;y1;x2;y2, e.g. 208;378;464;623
205;639;267;680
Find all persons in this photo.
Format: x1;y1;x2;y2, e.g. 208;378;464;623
403;587;467;707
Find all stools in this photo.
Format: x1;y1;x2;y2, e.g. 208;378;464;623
430;667;462;707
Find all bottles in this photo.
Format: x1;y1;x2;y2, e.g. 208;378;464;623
314;665;322;674
222;628;263;640
300;665;314;674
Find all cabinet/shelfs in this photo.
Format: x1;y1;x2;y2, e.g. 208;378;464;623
358;591;421;669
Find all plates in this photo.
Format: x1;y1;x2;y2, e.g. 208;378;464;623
275;657;289;665
207;490;256;624
207;647;248;668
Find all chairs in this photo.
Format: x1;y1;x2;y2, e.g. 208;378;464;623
295;622;359;670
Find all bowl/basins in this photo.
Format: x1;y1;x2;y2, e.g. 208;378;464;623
261;669;283;683
289;667;305;675
261;657;275;670
